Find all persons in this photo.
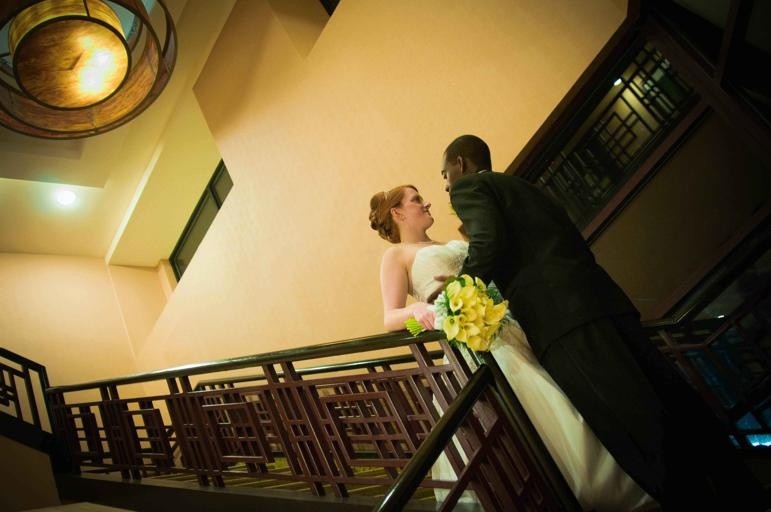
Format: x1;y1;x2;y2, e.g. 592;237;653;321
369;184;654;512
427;134;755;512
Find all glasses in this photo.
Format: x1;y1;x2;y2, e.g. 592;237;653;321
0;0;179;138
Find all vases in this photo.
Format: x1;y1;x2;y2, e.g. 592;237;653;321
402;274;510;352
